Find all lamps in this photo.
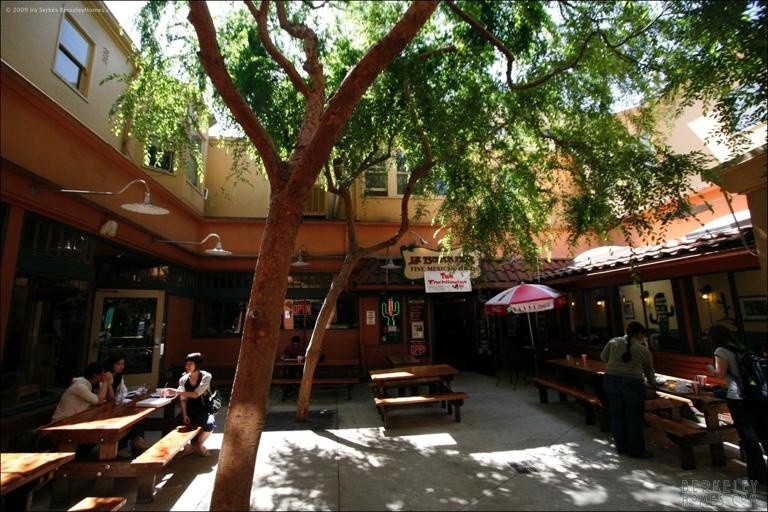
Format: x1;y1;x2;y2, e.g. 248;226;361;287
408;230;428;246
701;285;714;300
381;246;400;270
290;249;313;267
29;175;170;217
157;235;231;258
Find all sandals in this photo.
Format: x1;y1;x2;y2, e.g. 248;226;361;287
193;446;209;456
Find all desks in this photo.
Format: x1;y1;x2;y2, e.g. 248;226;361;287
0;452;75;496
37;389;176;497
367;364;459;419
203;363;239;369
546;358;740;468
274;358;359;368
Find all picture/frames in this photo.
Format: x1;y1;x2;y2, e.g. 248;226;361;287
740;293;768;322
623;300;635;320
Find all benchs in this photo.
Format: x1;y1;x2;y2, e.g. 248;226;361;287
270;376;360;401
133;420;206;502
372;392;470;433
368;378;444;398
213;379;232;387
67;496;128;512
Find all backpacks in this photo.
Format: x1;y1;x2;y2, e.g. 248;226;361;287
719;344;768;401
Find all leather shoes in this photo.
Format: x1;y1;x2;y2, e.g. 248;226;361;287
617;449;654;459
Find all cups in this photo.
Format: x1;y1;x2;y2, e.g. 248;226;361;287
160;388;168;397
699;376;707;386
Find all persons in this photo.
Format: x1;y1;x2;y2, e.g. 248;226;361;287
167;353;212;458
52;355;149;457
600;322;657;458
280;336;302;361
705;325;768;489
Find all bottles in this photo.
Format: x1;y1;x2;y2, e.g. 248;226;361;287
747;377;757;385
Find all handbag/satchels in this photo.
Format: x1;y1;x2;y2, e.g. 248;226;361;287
208;389;222;414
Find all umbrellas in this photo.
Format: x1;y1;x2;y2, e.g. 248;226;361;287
484;282;565;373
